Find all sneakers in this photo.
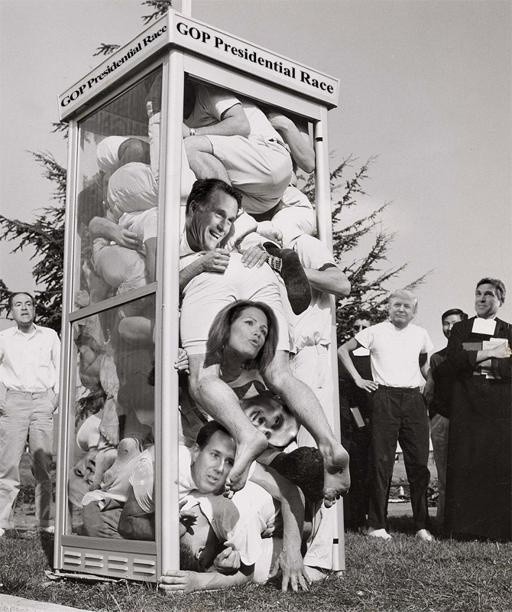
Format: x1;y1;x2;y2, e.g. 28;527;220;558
368;528;392;540
416;529;437;542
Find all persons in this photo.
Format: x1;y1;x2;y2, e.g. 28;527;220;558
337;312;378;531
425;309;469;537
337;290;437;543
0;292;61;538
70;62;351;594
445;278;512;538
